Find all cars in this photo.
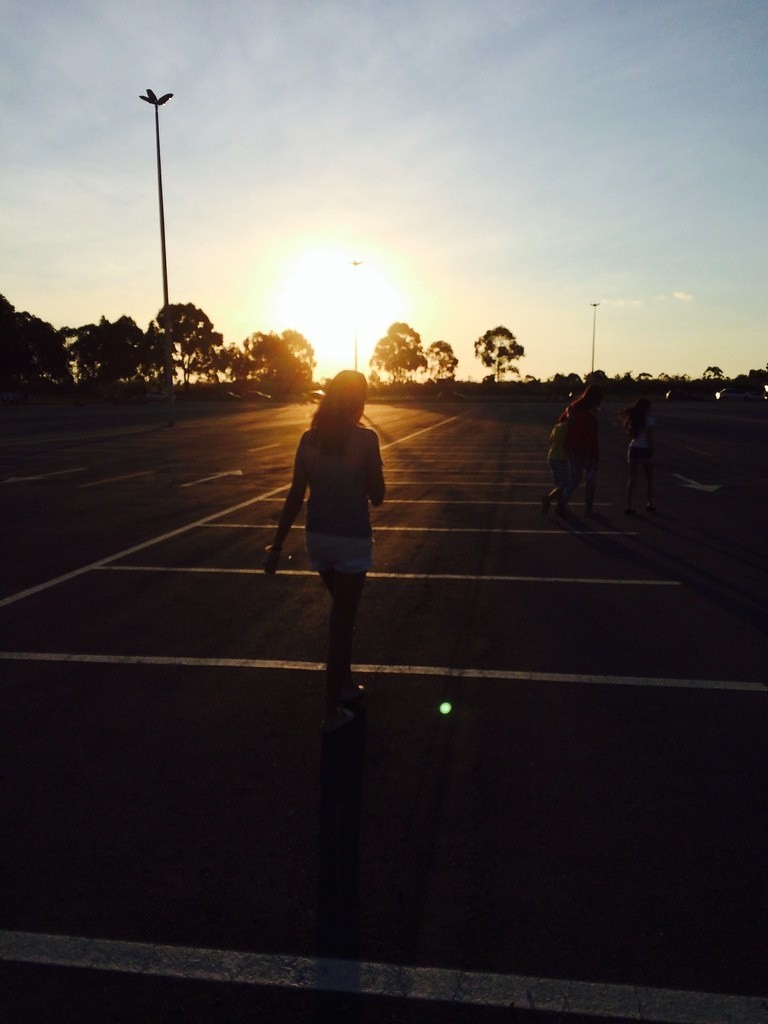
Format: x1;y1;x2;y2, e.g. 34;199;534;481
718;388;751;401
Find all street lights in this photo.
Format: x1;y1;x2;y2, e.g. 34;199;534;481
589;303;602;370
136;88;174;302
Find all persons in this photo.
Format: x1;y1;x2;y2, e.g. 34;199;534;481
262;367;385;737
617;396;658;519
540;417;579;517
556;381;604;525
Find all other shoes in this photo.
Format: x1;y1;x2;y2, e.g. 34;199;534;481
319;707;354;732
338;685;359;702
541;494;551;515
584;510;600;518
555;506;570;515
646;503;656;511
624;508;635;515
